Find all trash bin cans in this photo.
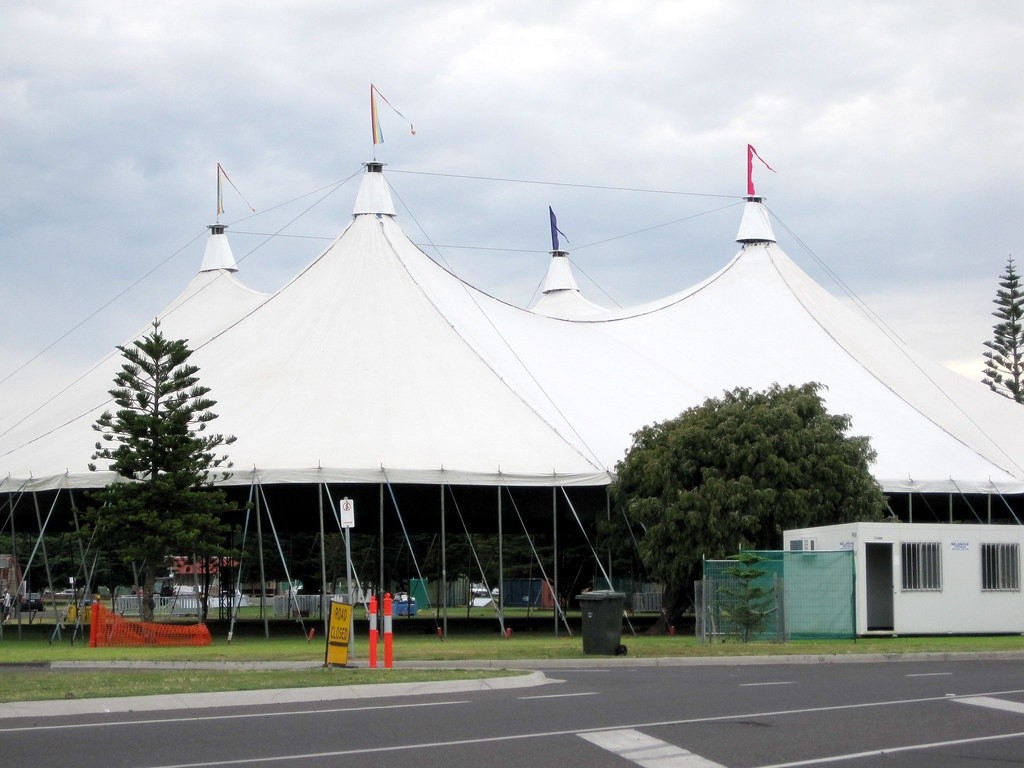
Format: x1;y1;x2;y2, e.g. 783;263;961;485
575;590;627;657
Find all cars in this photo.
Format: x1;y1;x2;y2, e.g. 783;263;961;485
21;593;43;611
471;582;499;597
55;589;77;597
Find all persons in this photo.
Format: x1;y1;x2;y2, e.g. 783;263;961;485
3;588;11;623
130;585;136;595
